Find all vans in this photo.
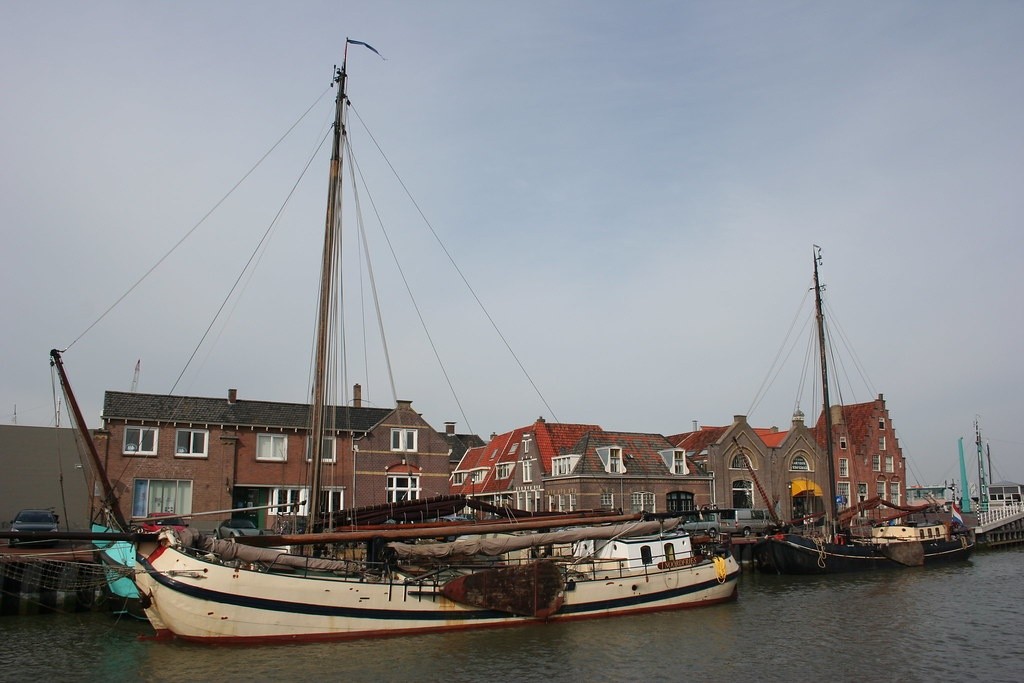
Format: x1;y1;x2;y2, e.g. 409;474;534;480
719;507;781;537
678;513;722;538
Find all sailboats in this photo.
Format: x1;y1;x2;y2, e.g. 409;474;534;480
41;36;746;650
688;240;979;576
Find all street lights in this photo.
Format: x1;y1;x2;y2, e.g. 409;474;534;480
785;479;795;521
468;473;480;521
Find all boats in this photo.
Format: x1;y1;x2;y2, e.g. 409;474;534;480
89;522;151;624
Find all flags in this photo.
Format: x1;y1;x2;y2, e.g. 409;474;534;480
953;503;963;524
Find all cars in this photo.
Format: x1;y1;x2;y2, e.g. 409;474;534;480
140;512;189;534
211;516;264;541
8;510;63;548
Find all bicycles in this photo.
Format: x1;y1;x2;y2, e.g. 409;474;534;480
270;511;293;534
